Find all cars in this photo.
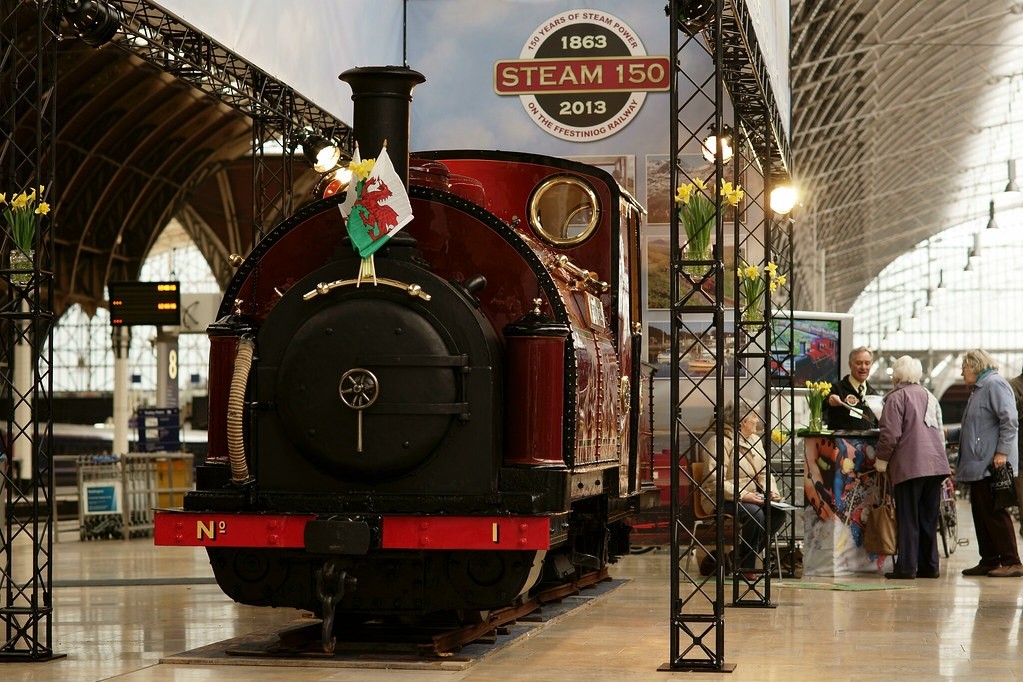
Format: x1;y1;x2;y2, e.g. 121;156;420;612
935;373;972;448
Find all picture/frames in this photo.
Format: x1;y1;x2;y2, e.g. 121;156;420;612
645;234;747;311
645;153;747;226
648;320;748;381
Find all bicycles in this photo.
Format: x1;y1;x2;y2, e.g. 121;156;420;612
936;444;969;558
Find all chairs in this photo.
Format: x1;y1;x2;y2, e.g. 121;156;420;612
684;463;734;579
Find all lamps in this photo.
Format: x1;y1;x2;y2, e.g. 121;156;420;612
1006;160;1020;192
298;134;341;172
62;0;120;48
701;122;734;166
987;200;999;228
965;234;981;270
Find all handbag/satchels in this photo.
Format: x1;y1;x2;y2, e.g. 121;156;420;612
757;482;763;494
862;503;898;555
987;460;1020;511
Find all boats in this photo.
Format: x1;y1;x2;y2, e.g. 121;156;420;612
688;355;716;368
657;348;690;359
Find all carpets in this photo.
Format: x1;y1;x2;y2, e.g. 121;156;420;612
695;577;918;592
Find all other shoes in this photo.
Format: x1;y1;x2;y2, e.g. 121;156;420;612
885;570;916;579
738;572;756;581
962;564;1000;576
916;571;940;578
729;550;741;573
987;564;1023;577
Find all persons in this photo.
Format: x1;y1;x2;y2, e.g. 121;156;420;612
701;397;786;582
873;355;952;579
1008;366;1023;536
956;348;1023;577
821;347;881;429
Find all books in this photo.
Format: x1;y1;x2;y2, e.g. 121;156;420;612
340;147;415;259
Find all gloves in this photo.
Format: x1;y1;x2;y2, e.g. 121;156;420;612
873;457;888;473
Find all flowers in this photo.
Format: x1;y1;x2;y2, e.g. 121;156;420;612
771;428;808;445
805;381;832;430
737;260;786;321
0;185;50;250
674;177;744;258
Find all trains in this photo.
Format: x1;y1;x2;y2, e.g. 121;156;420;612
154;63;663;654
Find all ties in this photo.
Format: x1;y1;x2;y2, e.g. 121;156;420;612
858;385;866;407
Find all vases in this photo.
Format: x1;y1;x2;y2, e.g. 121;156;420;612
809;413;823;432
743;313;763;331
684;251;712;280
10;250;35;285
